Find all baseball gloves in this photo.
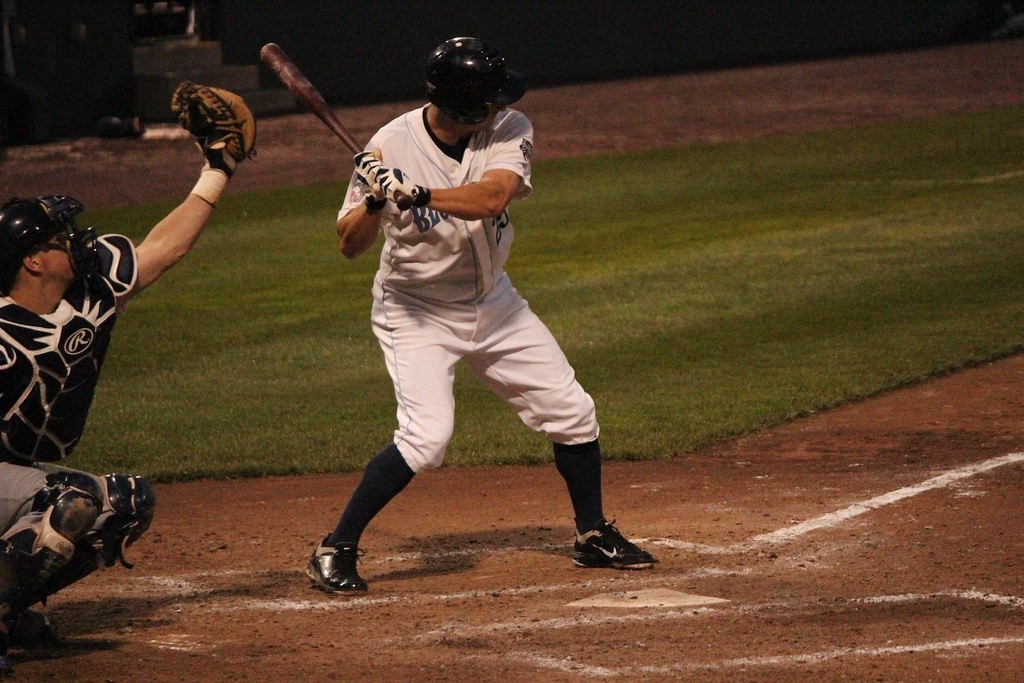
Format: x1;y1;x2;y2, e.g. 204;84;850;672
165;79;259;181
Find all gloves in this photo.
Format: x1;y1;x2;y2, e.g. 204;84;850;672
352;151;432;212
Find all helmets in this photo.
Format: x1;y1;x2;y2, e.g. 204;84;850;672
425;36;529;119
1;197;67;295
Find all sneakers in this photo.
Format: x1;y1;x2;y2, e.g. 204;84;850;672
304;530;370;597
570;518;656;570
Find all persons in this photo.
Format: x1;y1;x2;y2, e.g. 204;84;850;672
0;83;256;669
304;37;655;595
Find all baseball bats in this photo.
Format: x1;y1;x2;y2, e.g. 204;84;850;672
258;42;415;211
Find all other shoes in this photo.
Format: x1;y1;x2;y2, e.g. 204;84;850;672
1;611;58;651
